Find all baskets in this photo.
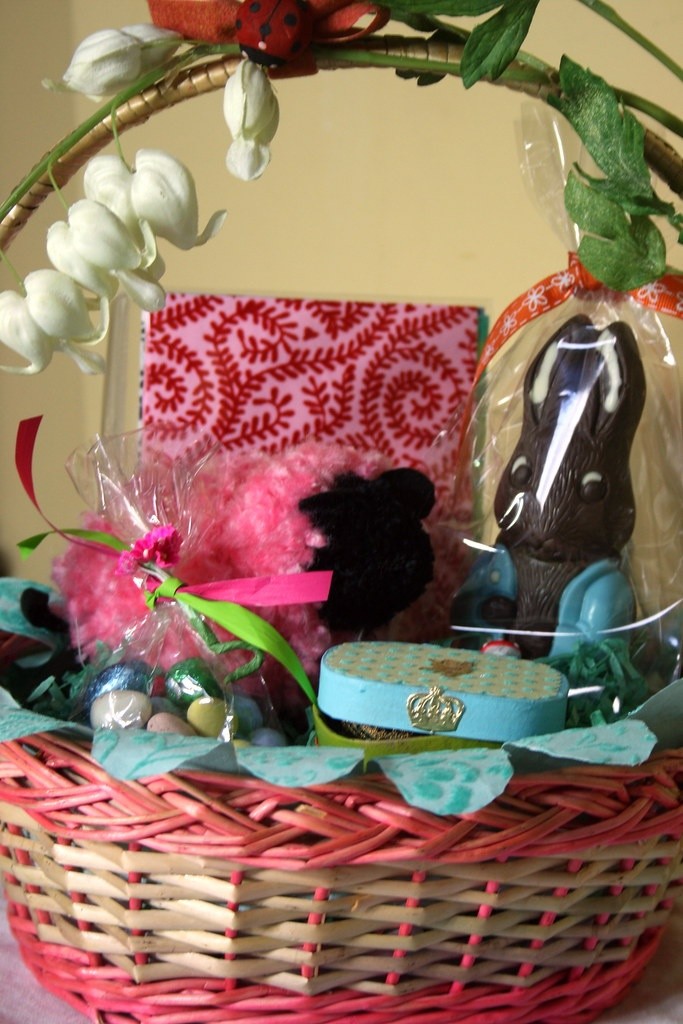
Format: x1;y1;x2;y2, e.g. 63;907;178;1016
0;36;683;1024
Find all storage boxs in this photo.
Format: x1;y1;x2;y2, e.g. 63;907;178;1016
312;642;569;772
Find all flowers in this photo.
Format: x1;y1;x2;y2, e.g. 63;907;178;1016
0;0;682;375
